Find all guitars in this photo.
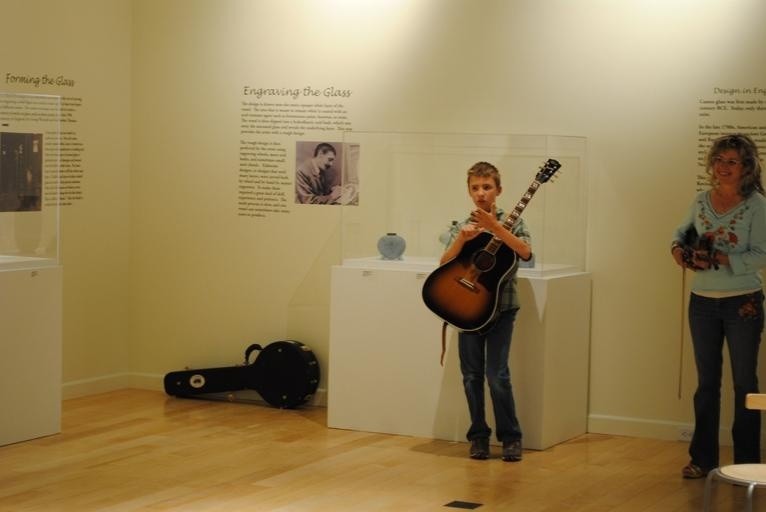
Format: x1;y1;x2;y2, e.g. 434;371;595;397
421;157;562;333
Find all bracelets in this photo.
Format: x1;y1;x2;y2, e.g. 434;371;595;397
671;244;681;255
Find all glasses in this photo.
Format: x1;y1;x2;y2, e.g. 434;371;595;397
711;157;741;166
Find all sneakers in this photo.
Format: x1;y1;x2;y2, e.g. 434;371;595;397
683;462;707;477
502;440;522;461
470;437;490;458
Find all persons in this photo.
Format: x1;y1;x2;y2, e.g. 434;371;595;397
668;133;766;487
293;143;347;205
438;161;535;462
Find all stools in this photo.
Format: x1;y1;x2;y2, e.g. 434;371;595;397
701;461;766;512
